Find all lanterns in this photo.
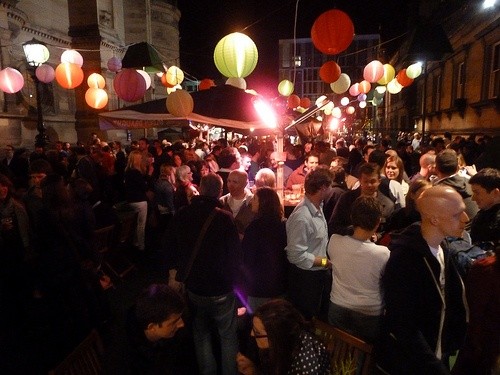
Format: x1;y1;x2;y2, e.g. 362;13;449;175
0;9;421;119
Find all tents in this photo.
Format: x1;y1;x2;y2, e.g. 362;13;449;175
101;84;323;140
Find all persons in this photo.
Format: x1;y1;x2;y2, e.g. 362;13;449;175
0;129;500;375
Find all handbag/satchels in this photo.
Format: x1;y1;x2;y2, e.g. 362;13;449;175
179;281;189;315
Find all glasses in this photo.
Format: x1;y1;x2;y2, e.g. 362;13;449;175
250;321;268;339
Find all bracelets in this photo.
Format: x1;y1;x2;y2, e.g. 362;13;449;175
322;258;327;267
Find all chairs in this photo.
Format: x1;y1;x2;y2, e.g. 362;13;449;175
311;315;374;375
